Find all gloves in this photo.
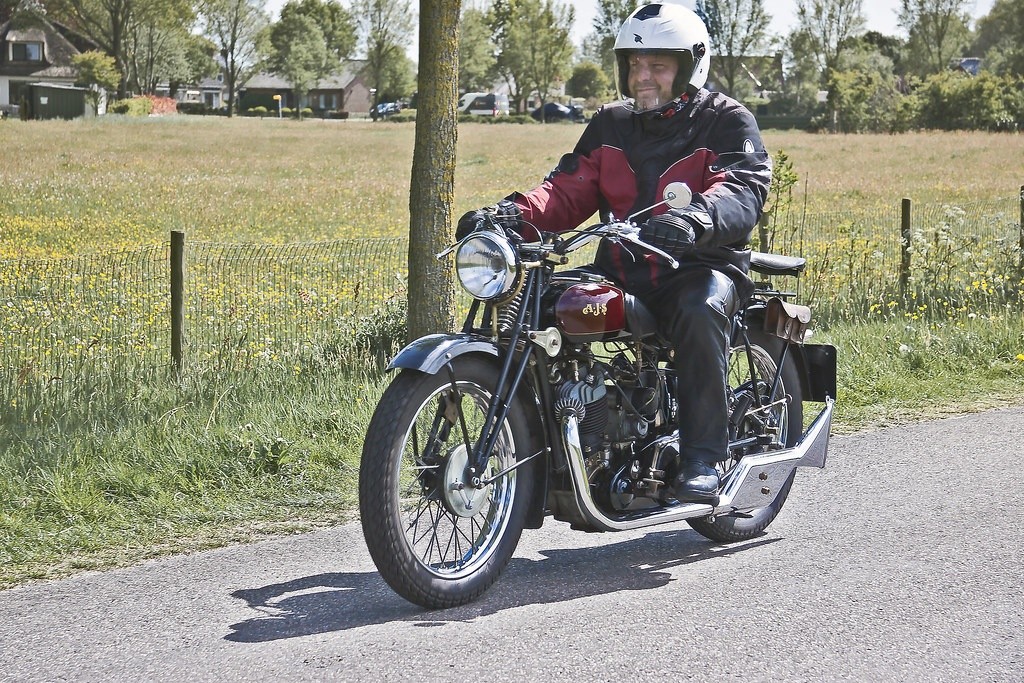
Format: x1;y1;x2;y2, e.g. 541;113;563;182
639;213;693;263
455;200;524;242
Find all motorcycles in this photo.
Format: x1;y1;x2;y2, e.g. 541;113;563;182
359;181;837;609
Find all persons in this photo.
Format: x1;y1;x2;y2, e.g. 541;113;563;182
455;4;772;500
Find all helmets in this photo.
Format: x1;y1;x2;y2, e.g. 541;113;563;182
612;3;710;98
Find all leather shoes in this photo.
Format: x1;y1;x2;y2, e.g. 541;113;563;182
674;456;720;507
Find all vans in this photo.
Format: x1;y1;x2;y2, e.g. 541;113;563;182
459;93;509;117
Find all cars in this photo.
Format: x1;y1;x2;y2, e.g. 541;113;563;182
370;103;401;118
